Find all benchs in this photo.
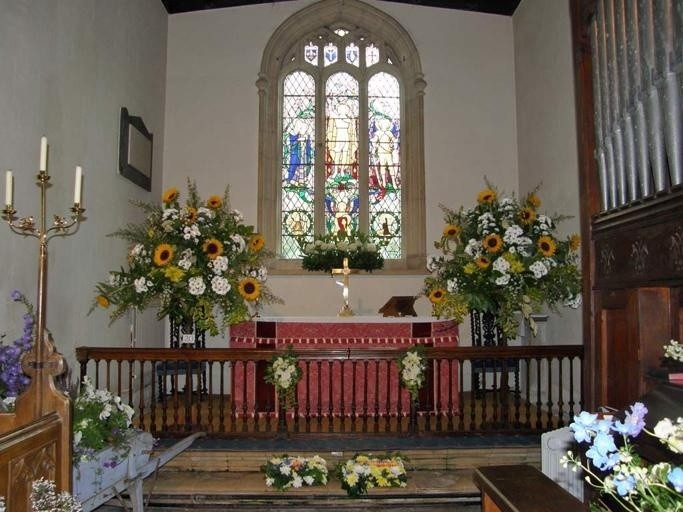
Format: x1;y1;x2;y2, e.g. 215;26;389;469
475;463;590;512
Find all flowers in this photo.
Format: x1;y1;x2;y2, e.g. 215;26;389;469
260;451;409;494
396;348;426;389
296;234;386;274
408;184;581;325
267;357;302;403
95;182;267;338
1;292;134;457
571;403;683;511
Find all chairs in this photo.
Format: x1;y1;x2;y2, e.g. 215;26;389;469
468;302;518;396
154;301;205;404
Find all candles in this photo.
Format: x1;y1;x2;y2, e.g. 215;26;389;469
74;165;82;204
40;136;47;171
5;169;12;210
